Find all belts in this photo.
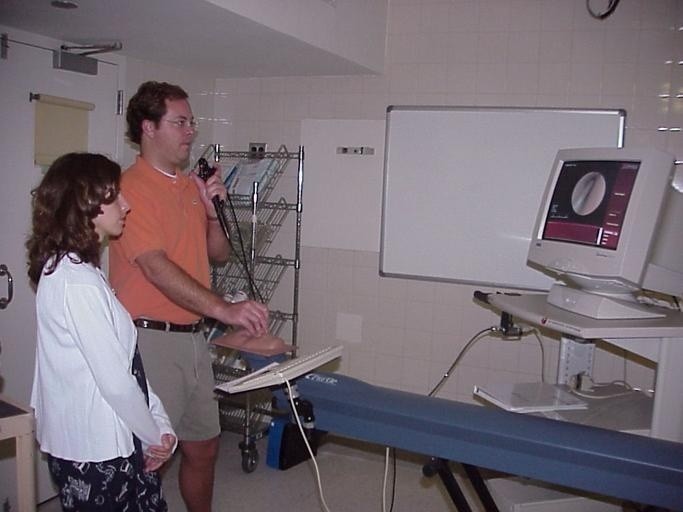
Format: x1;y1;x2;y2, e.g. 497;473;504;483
134;317;204;334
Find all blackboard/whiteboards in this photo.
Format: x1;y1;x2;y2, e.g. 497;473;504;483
378;105;626;292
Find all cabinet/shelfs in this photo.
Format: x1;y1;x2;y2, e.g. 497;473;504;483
472;290;682;512
189;144;305;473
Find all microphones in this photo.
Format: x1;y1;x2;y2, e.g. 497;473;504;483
197;157;230;240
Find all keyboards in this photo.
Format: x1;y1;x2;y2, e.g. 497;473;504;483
228;343;344;395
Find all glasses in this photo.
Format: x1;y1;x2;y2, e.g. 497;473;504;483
163;117;200;129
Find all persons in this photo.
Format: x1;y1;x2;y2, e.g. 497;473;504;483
109;82;270;512
24;152;179;512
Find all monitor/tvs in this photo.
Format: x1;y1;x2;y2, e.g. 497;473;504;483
526;147;683;320
